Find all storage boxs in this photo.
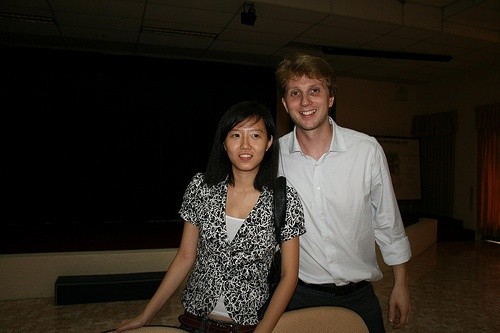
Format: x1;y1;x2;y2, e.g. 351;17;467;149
54;271;166;305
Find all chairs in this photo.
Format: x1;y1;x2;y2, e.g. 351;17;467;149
255;305;369;333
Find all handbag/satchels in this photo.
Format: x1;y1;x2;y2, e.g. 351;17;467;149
268;175;287;289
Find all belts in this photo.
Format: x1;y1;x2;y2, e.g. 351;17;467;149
177;308;257;333
296;278;370;296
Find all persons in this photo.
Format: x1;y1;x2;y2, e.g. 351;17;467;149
114;100;306;333
273;54;412;333
